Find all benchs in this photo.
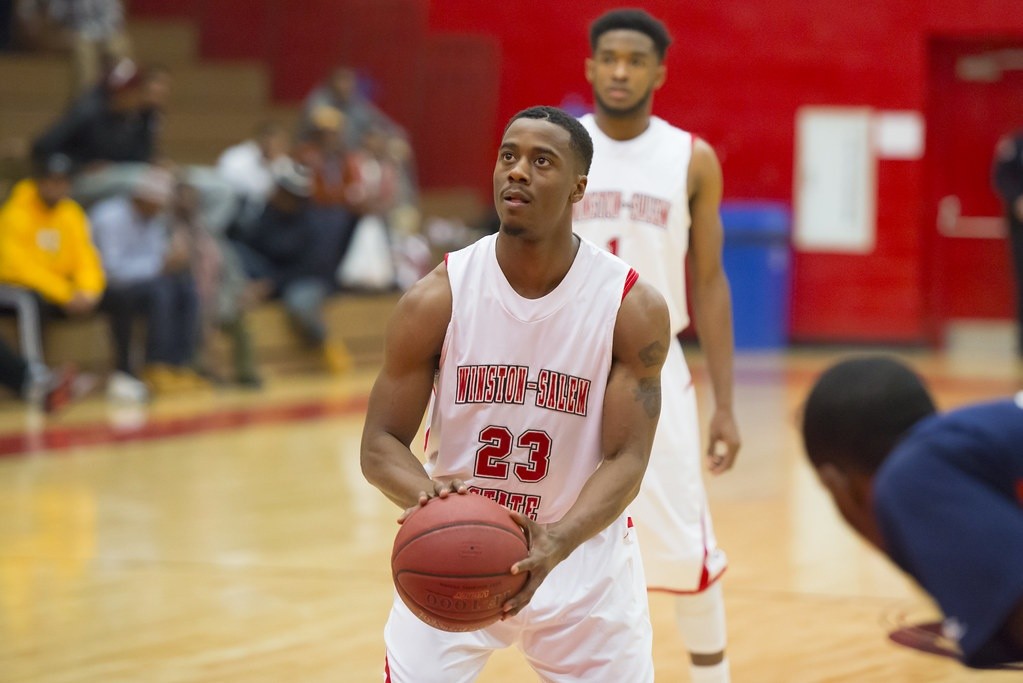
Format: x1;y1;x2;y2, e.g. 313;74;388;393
0;26;452;373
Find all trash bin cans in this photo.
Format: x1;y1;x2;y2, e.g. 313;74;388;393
720;205;791;350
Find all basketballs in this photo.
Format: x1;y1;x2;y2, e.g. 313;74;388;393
388;492;530;633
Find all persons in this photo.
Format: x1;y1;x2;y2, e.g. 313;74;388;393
572;13;743;682
803;356;1022;670
994;133;1022;351
356;107;670;683
0;0;432;404
